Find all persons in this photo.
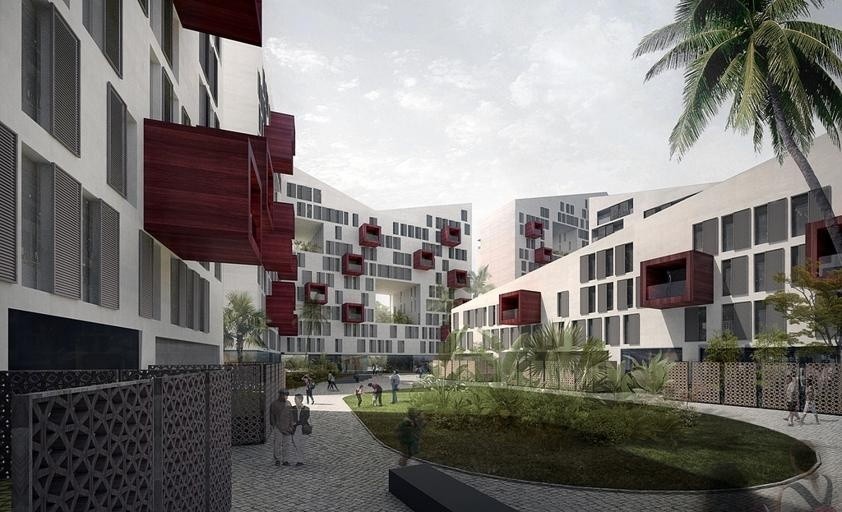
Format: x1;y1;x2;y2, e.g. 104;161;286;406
355;384;363;407
702;465;775;511
371;363;376;375
301;374;315;405
269;388;293;468
327;370;340;392
389;368;400;404
782;375;821;427
780;440;836;512
369;392;377;407
397;407;427;468
368;382;383;407
290;393;312;467
664;270;673;299
